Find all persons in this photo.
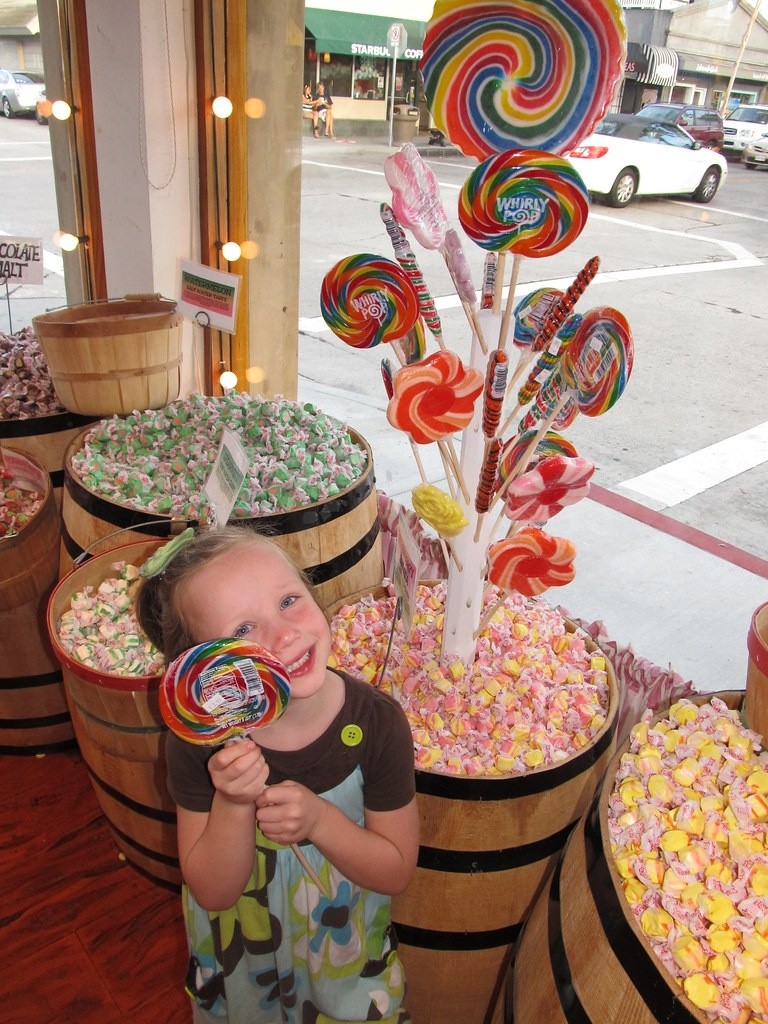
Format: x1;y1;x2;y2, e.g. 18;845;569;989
137;525;421;1023
302;84;322;138
313;83;335;139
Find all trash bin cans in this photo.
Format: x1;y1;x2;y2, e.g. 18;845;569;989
388;104;419;147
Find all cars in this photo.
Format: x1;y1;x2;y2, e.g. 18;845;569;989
740;132;768;170
565;114;728;209
35;89;49;124
0;69;46;119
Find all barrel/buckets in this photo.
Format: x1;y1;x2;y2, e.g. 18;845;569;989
45;537;185;898
57;395;385;610
0;443;79;754
0;413;107;526
320;578;623;1023
484;686;768;1024
742;601;768;753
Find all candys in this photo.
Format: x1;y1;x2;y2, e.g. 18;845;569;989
158;638;291;747
322;0;633;596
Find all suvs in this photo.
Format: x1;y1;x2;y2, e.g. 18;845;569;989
720;102;768;155
635;101;725;154
